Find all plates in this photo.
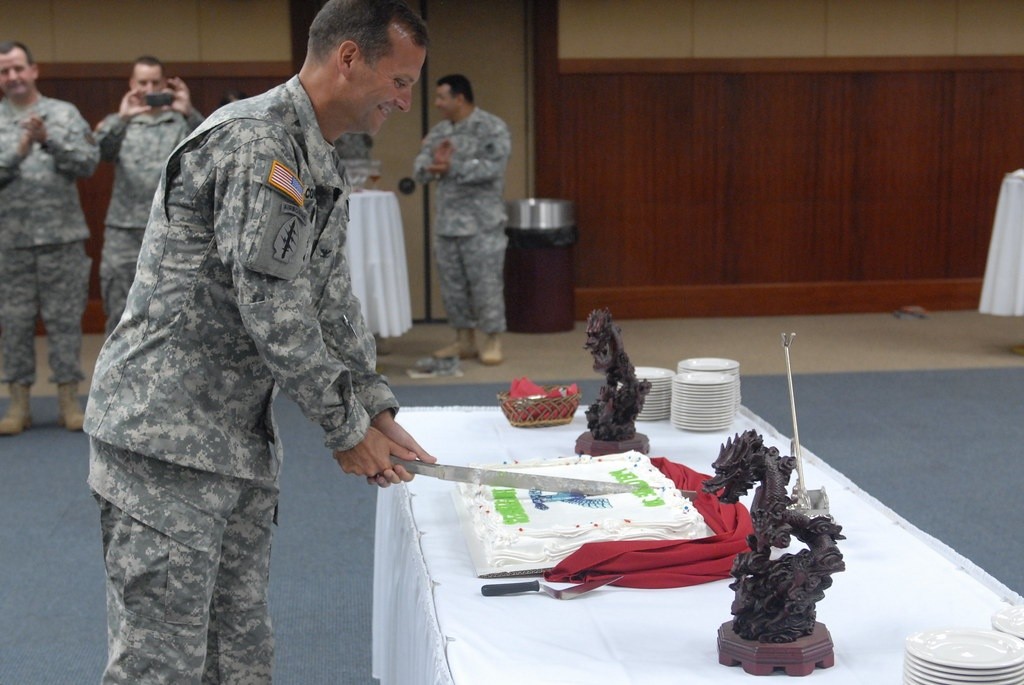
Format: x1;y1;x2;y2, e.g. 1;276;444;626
902;603;1024;685
677;357;741;416
616;366;676;421
670;372;735;430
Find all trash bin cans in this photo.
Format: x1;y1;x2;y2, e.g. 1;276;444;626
503;198;578;334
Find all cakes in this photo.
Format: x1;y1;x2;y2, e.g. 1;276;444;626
449;449;707;577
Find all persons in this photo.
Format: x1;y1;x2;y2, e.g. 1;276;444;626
0;40;102;435
410;74;514;365
84;1;438;685
95;54;204;340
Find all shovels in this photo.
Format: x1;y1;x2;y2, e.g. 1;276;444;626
480;574;623;601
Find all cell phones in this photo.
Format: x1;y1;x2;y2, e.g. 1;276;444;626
144;91;174;107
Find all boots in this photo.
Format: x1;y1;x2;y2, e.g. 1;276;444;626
0;382;33;436
57;383;85;431
432;328;479;360
478;333;504;367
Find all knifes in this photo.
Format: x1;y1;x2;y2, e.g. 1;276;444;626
388;454;700;501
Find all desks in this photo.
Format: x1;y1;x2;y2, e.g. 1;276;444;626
372;406;1024;685
978;171;1024;356
345;189;413;355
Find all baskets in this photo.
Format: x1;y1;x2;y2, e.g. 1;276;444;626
495;384;584;430
